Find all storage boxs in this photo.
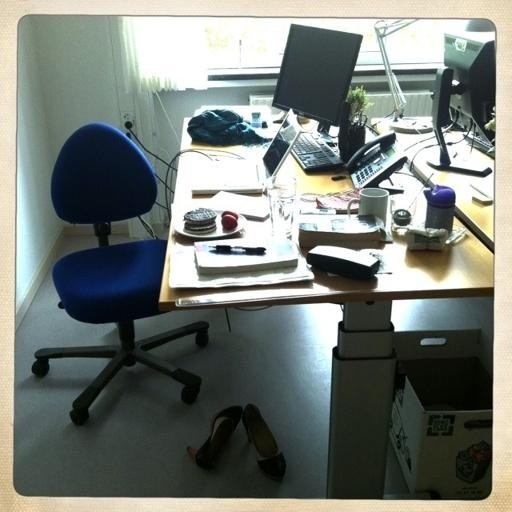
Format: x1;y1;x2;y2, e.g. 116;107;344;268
389;328;493;498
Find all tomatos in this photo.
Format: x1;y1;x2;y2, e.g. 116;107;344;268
222;211;238;220
222;215;237;229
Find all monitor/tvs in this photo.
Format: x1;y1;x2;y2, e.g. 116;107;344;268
425;31;495;178
272;23;363;146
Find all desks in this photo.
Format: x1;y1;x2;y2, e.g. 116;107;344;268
157;105;494;498
372;117;494;250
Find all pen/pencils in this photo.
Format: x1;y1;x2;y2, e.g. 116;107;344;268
208;245;266;252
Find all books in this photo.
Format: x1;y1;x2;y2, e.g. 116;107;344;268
193;234;299;277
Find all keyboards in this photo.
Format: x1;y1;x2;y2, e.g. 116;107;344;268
463;131;495;158
289;130;344;172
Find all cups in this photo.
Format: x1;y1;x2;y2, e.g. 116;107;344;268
346;188;389;229
266;176;297;242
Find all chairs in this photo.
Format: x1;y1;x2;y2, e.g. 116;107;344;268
31;122;209;424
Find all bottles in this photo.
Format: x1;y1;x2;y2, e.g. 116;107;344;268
423;184;456;237
251;112;261;129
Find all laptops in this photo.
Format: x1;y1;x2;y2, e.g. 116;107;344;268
191;108;302;195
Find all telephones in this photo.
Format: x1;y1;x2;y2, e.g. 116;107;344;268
346;132;408;191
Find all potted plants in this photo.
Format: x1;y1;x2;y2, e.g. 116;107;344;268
338;84;374;162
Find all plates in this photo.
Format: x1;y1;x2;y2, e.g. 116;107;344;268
172;209;248;239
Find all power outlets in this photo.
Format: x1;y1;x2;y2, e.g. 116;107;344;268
120;111;137;143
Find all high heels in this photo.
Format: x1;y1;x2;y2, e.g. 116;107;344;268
187;405;242;470
242;403;286;482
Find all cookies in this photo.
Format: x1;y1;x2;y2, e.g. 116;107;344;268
184;208;216;234
